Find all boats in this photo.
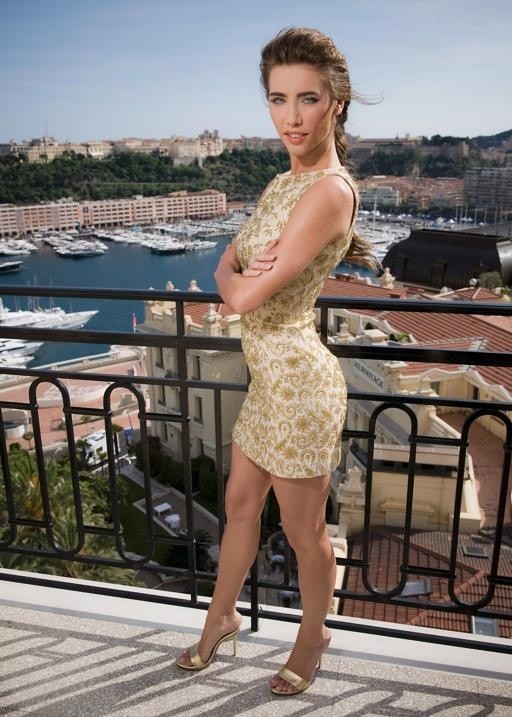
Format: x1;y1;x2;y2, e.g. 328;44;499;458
0;275;99;380
354;209;474;265
0;206;256;276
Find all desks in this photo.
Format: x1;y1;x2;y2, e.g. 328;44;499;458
153;501;173;517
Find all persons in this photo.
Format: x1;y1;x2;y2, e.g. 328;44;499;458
176;28;383;695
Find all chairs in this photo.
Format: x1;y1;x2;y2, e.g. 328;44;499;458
164;513;181;528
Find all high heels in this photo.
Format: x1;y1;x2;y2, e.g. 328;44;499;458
175;628;239;670
270;648;321;695
